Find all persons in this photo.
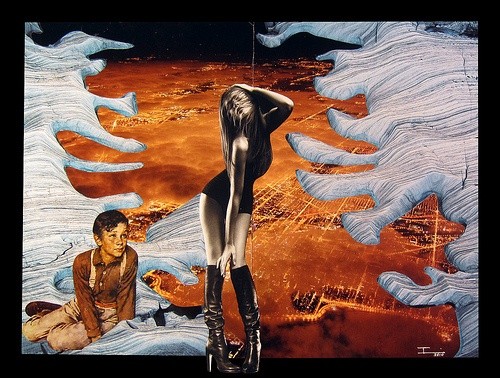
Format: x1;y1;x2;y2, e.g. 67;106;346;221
196;83;295;376
